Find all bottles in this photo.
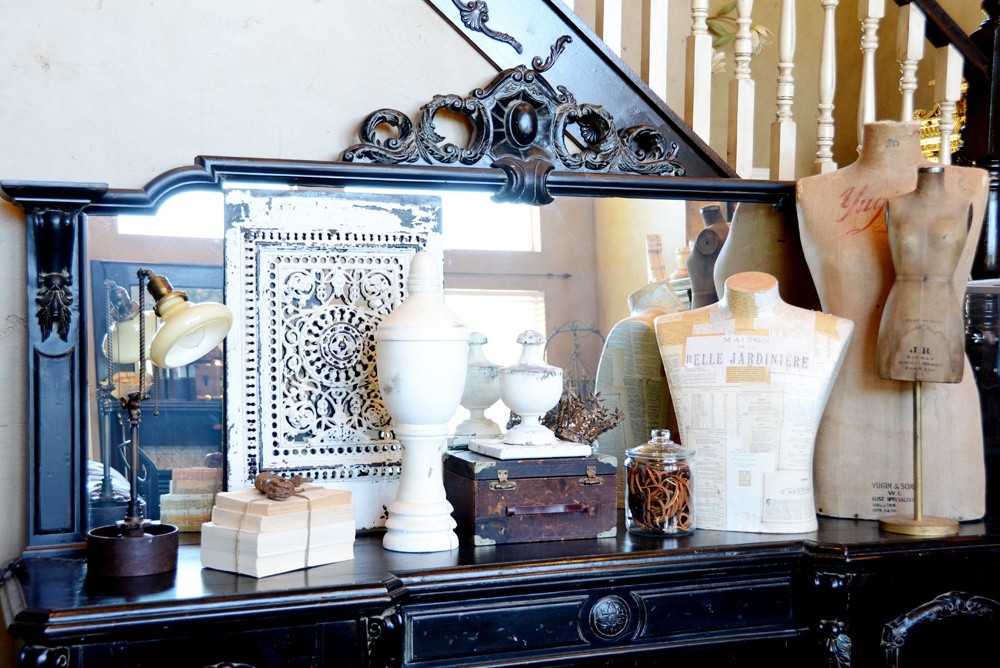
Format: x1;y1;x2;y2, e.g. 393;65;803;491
623;430;696;538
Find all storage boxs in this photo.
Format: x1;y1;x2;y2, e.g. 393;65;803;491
444;449;617;547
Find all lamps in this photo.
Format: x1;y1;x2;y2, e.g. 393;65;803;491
89;279;147;530
88;268;234;577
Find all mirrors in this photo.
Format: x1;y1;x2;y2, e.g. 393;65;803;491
75;175;823;534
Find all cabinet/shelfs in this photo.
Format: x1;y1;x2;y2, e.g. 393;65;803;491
0;65;1000;668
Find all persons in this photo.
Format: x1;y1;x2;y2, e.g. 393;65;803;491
875;165;974;384
795;120;989;521
687;205;730;309
592;273;690;509
654;270;854;536
